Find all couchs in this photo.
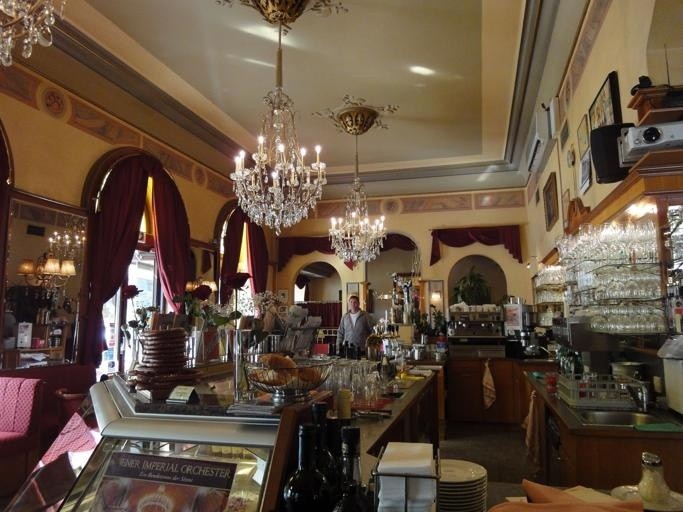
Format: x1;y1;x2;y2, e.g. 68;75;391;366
8;363;94;436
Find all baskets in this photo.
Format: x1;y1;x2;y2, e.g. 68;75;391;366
242;351;334;398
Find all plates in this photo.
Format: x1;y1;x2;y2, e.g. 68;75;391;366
610;483;682;512
433;458;489;511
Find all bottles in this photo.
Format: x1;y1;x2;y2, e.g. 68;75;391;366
665;282;683;334
381;356;389;378
639;453;670;511
559;351;583;382
336;388;352;420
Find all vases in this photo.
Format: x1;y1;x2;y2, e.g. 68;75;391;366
130;327;295;404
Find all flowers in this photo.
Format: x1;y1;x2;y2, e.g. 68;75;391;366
121;273;308;330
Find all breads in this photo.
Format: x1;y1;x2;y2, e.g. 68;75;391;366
251;352;321;388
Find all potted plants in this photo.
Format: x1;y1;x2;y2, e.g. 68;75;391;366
417;315;429;343
430;304;447;336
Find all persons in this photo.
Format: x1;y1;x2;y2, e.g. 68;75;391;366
335;295;374;359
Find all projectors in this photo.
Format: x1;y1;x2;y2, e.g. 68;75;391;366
620;121;682;164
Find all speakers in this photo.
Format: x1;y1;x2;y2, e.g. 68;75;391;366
590;123;636;184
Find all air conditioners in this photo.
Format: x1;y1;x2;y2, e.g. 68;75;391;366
523;110;554;176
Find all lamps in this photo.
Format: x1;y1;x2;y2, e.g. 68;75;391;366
46;217;86;260
0;0;66;67
18;258;47;286
215;0;351;236
313;95;399;264
44;259;77;291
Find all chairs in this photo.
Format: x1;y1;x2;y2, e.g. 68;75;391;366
1;377;45;495
54;387;90;426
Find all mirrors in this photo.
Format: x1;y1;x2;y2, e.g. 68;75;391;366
5;188;93;368
421;280;445;324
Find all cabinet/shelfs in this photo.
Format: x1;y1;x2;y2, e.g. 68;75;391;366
4;357;332;512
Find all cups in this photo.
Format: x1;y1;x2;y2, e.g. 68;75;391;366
556;221;663;335
318;355;382;401
535;265;565;302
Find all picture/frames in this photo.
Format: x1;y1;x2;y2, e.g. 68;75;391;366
589;70;623;130
